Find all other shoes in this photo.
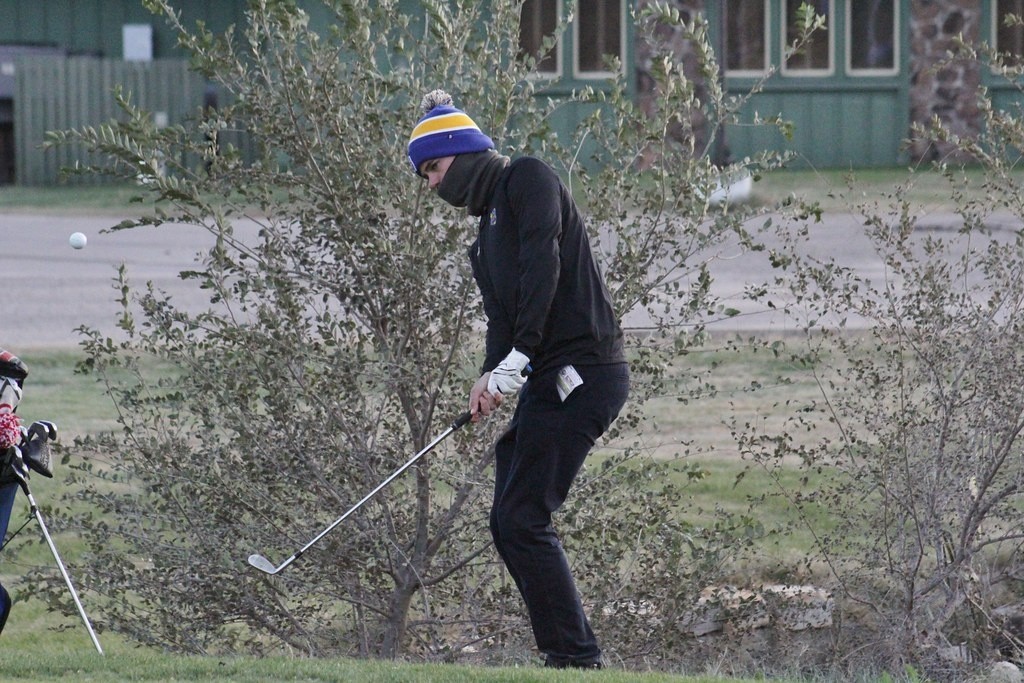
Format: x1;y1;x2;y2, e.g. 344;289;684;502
544;659;601;670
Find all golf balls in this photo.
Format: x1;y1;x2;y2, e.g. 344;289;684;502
70;232;86;249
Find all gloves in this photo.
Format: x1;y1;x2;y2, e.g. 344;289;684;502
487;347;531;402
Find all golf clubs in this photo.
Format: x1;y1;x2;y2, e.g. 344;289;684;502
2;420;57;466
248;365;532;575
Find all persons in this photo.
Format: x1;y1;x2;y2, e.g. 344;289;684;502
408;106;632;669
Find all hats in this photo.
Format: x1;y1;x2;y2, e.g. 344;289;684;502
408;89;495;177
